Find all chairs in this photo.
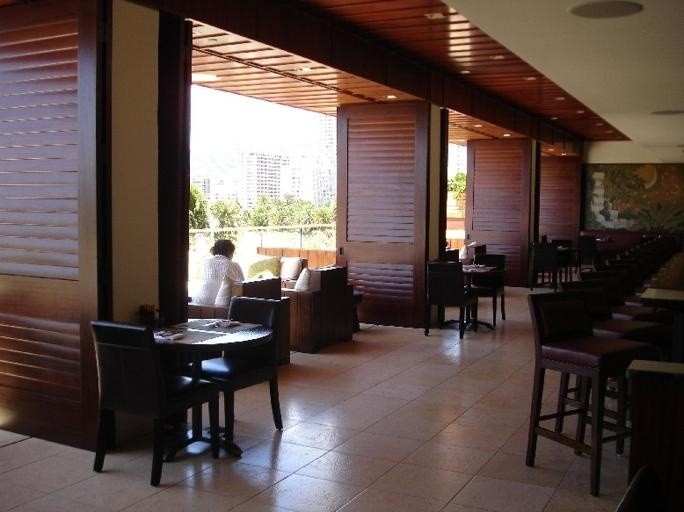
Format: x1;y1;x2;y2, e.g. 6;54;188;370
425;259;478;339
187;296;229;318
187;293;283;447
471;254;506;326
282;266;354;352
525;231;684;497
89;318;220;485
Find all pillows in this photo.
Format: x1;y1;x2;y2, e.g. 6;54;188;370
281;259;301;281
295;267;310;292
248;257;280;282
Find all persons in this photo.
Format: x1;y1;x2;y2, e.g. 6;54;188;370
191;239;244;305
